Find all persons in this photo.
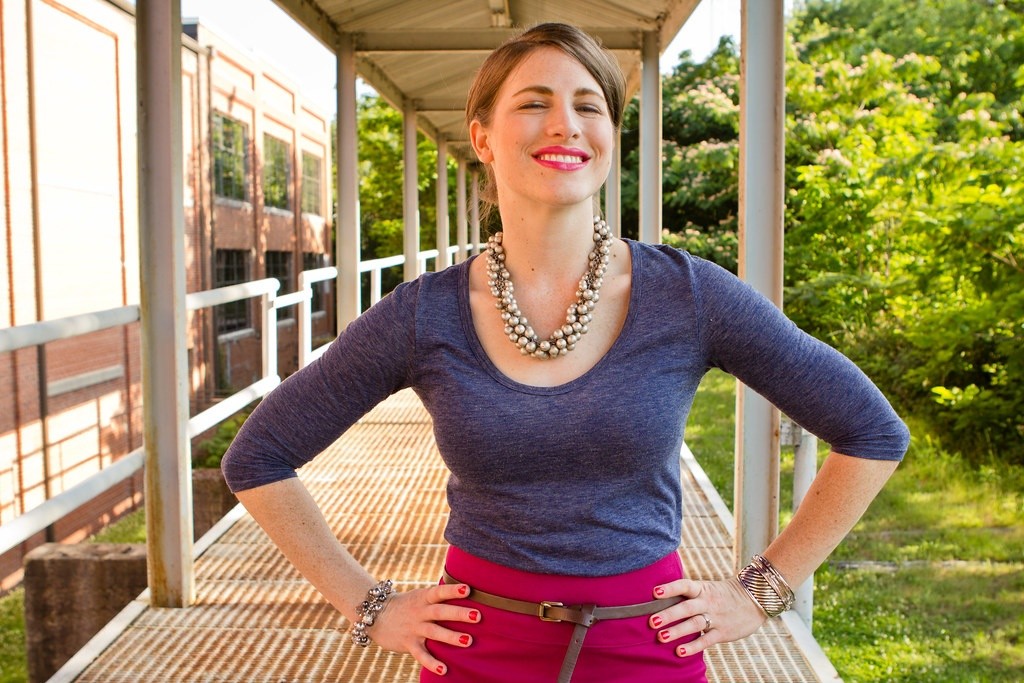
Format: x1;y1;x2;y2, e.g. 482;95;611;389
219;20;911;683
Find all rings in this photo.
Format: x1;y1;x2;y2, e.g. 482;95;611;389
702;613;711;634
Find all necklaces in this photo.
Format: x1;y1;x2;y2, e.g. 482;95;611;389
485;214;613;360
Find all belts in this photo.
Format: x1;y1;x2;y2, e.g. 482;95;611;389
443;566;686;683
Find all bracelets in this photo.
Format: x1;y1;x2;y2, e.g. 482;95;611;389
351;579;393;649
736;555;798;619
